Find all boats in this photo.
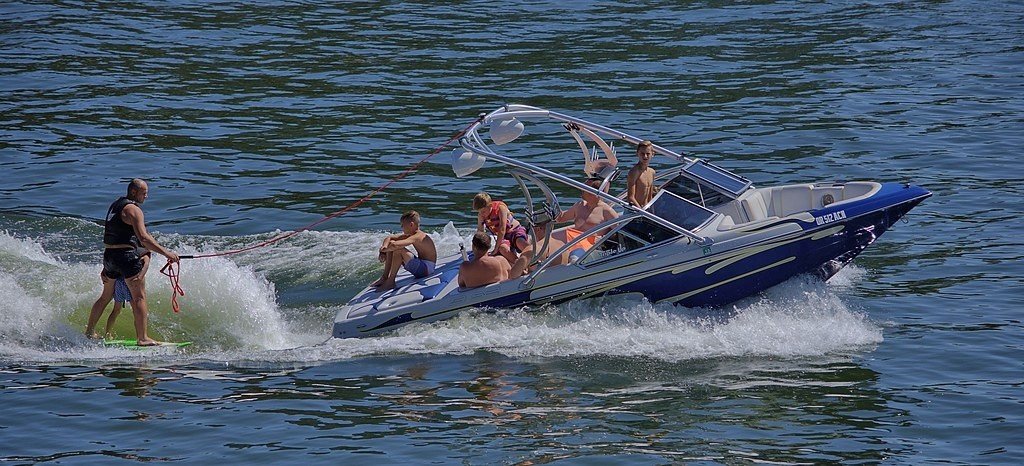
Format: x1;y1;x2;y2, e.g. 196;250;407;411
330;103;936;341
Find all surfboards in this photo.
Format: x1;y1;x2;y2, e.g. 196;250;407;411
99;339;193;352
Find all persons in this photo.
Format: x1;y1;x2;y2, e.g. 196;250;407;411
623;141;655;215
370;210;437;292
85;180;180;346
101;241;152;341
458;177;619;289
